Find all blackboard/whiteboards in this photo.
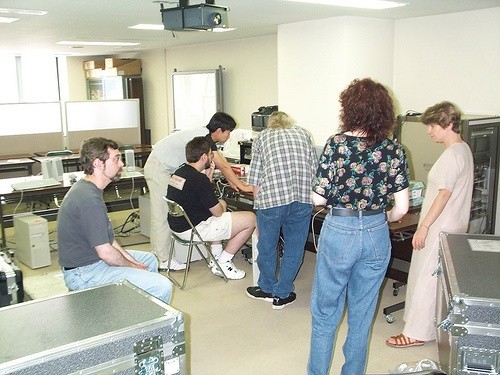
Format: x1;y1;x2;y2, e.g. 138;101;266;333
172;65;223;131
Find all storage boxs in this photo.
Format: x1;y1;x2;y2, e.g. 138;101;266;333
0;252;24;307
0;279;188;375
434;230;500;375
83;58;141;76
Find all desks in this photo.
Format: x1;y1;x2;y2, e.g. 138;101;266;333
213;183;423;323
0;143;155;249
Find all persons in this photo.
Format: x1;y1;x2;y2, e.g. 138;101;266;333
57;138;172;305
166;135;257;280
246;110;318;309
386;102;473;348
307;77;410;375
143;111;254;272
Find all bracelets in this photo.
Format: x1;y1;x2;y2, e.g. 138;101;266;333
421;225;429;229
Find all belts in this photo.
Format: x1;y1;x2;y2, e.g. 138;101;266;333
330;206;382;217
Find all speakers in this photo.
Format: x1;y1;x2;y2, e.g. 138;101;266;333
41;158;64;181
125;150;135;172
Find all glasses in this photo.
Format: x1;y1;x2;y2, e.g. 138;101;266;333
210;152;214;159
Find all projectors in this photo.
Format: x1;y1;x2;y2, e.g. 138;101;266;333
162;3;230;31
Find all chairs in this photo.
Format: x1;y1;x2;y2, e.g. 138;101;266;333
162;195;227;289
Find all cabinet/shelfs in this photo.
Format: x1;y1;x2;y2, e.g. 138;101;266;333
86;74;146;143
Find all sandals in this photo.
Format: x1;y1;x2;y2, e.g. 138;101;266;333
386;333;424;348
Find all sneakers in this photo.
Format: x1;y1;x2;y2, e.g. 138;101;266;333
212;260;246;280
245;285;273;302
272;291;297;309
208;254;235;268
158;257;190;271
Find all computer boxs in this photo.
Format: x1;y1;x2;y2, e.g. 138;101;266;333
138;194;151;239
13;213;52;270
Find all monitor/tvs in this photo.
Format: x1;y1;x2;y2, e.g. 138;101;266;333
251;105;279;131
